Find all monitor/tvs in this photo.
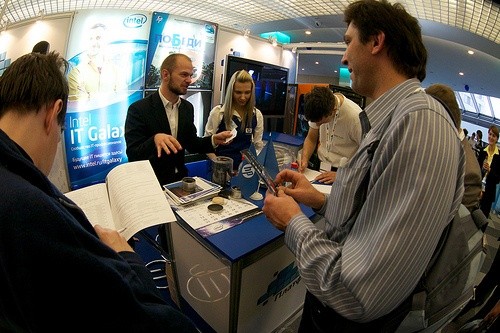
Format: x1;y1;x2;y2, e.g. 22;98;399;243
221;54;289;119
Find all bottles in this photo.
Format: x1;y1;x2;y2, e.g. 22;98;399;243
285;162;298;187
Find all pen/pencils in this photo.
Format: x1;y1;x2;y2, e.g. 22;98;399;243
298;152;303;175
240;148;279;196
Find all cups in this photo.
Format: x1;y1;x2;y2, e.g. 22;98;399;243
182;177;196;192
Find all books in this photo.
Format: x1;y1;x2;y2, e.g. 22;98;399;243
163;175;223;208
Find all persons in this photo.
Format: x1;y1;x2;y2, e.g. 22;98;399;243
263;0;468;333
296;86;365;184
203;68;263;166
124;54;234;190
422;84;489;234
463;120;500;219
0;51;200;333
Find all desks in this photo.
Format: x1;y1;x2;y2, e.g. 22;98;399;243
165;163;333;333
262;130;305;156
